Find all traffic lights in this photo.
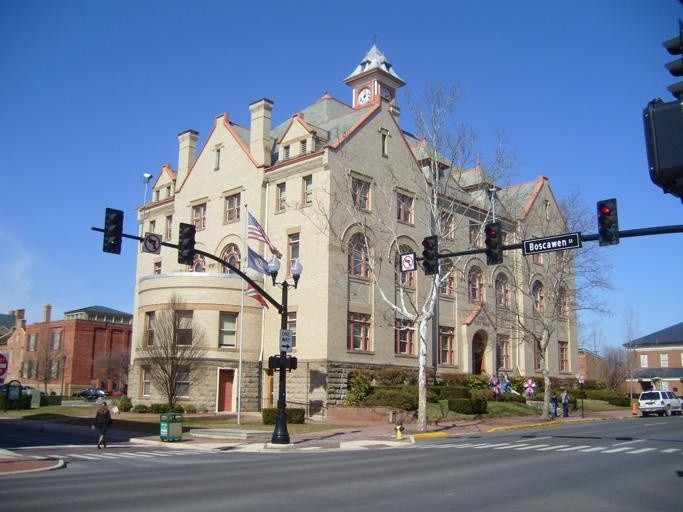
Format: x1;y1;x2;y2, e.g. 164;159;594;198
482;220;505;268
266;352;278;370
597;198;619;249
176;220;196;265
422;233;442;276
660;17;682;104
642;97;682;204
283;357;298;372
101;206;123;255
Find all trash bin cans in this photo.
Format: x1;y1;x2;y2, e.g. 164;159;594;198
160;413;183;442
388;410;397;424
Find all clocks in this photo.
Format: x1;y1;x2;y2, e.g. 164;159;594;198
354;80;395;110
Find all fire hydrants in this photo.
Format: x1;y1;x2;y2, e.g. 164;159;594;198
392;419;404;440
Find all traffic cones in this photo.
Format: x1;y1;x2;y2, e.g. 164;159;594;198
631;402;638;415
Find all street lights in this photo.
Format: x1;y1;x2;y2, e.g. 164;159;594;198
263;255;306;450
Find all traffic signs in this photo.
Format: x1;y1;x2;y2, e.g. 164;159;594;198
522;233;581;257
278;329;292;354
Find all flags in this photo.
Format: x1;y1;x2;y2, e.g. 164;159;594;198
245;282;268;311
246;212;283;259
247;246;271;276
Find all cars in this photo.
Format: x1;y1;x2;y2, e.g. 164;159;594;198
0;378;123;410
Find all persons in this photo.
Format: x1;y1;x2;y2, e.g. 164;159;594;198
550;391;558;418
560;390;569;418
91;402;112;449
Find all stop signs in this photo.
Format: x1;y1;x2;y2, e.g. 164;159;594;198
0;351;9;379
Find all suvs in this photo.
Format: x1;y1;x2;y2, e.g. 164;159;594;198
636;391;681;418
